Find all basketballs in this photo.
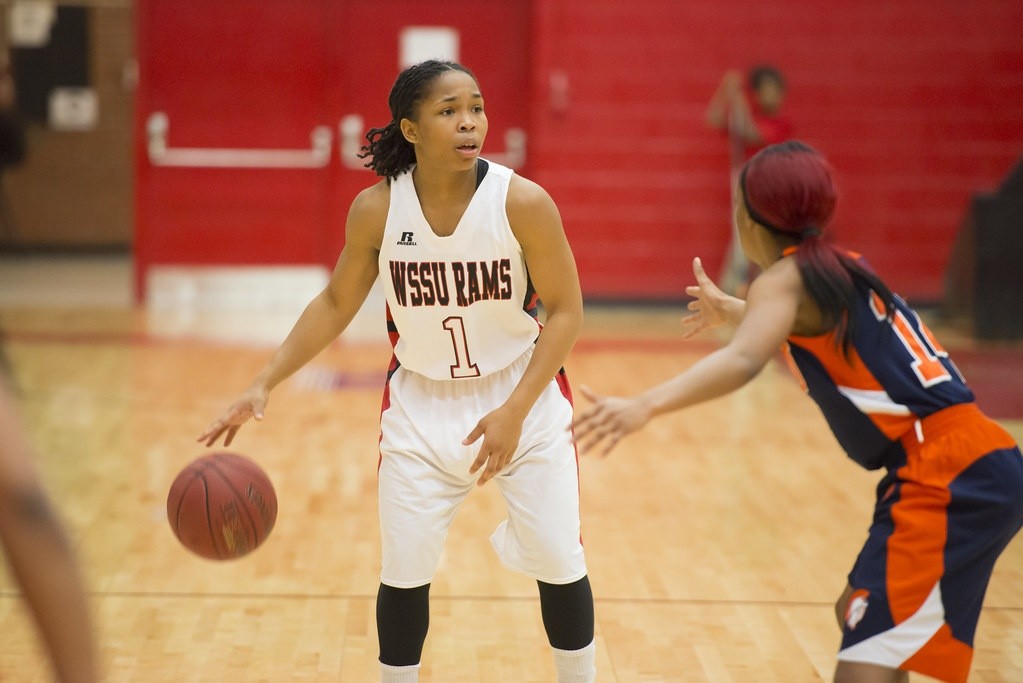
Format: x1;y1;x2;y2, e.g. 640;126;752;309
165;451;279;562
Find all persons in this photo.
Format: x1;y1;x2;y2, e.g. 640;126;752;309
705;67;789;290
566;142;1023;682
197;59;595;683
0;413;101;683
